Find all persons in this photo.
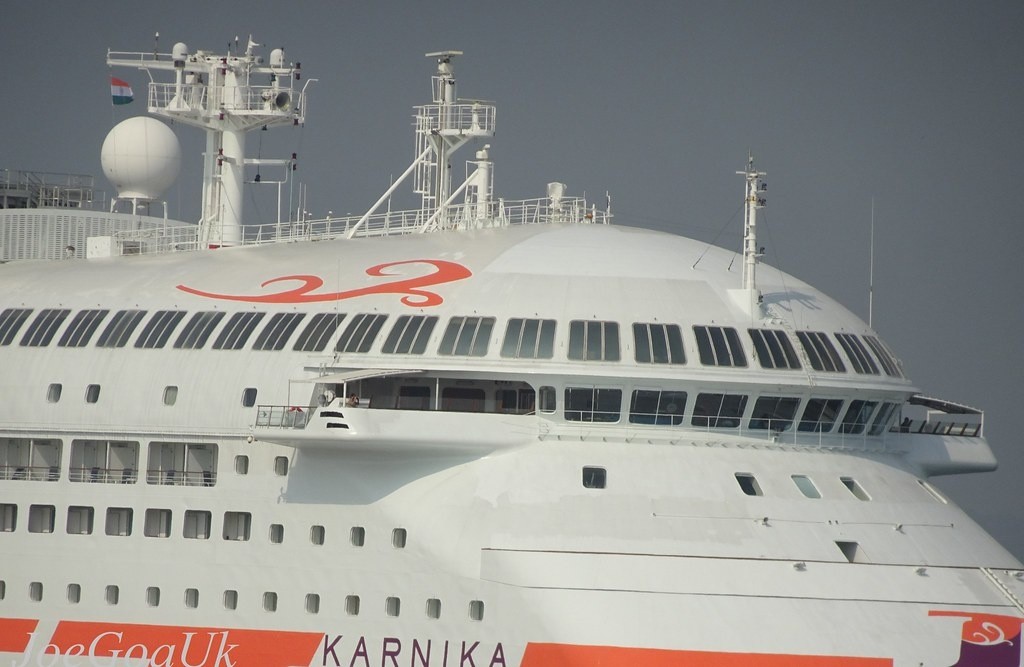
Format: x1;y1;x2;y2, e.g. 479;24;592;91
347;394;359;407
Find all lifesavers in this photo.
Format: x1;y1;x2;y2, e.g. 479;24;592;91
288;407;303;412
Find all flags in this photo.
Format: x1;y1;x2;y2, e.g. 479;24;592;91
111;76;134;105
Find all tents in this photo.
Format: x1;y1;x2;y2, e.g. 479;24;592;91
284;369;427;425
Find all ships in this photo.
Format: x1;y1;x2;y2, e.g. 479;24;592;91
0;36;1024;667
